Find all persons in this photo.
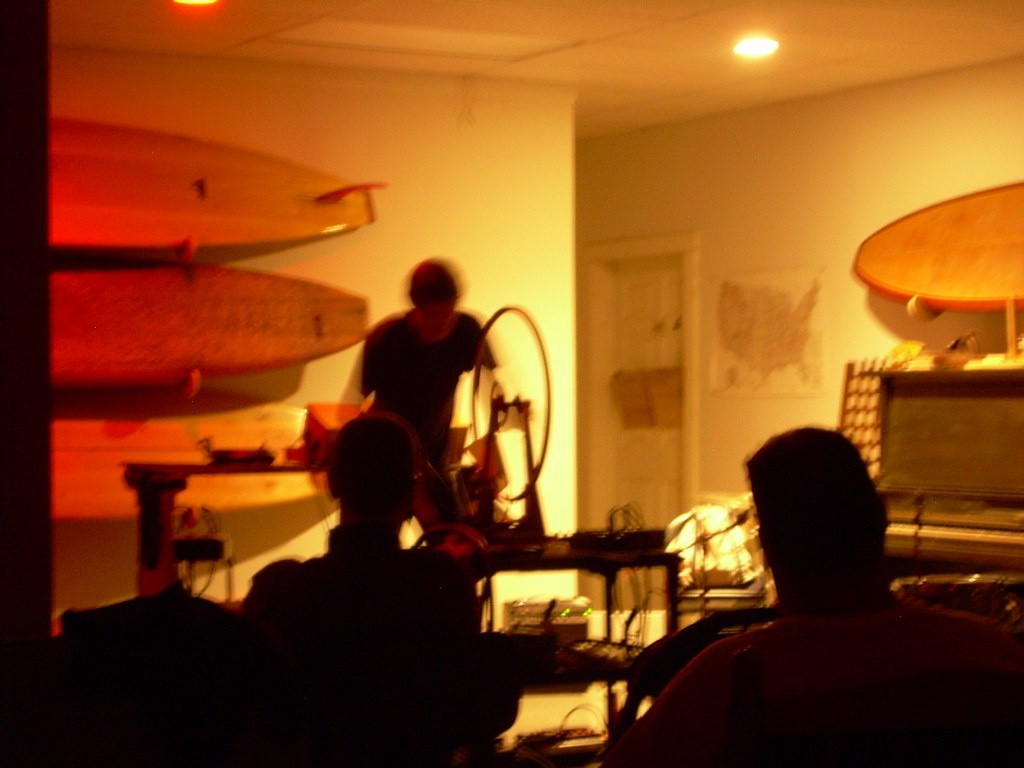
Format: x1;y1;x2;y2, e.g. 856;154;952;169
55;575;305;767
233;408;526;768
595;426;1024;768
363;262;486;516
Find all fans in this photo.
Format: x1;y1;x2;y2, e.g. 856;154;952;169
471;307;551;543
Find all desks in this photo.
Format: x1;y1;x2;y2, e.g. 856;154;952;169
119;459;326;596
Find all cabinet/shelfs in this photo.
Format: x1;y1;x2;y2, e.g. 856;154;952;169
424;552;682;768
